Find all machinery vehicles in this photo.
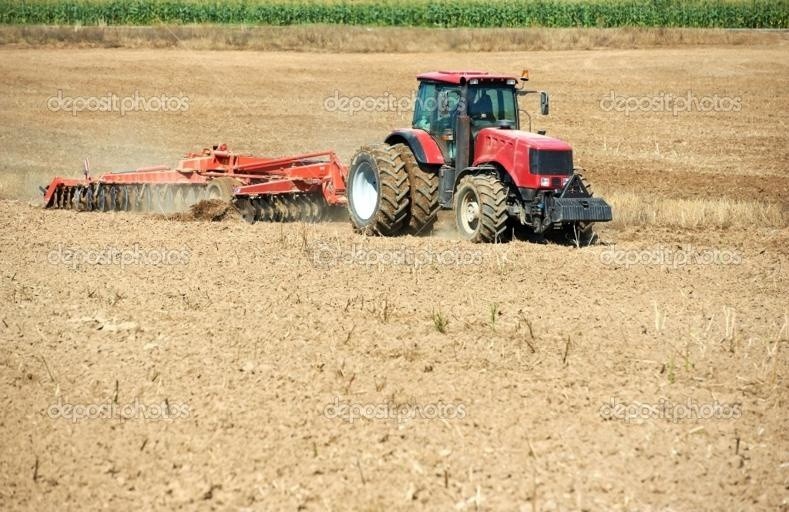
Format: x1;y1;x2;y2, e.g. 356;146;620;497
40;66;614;246
